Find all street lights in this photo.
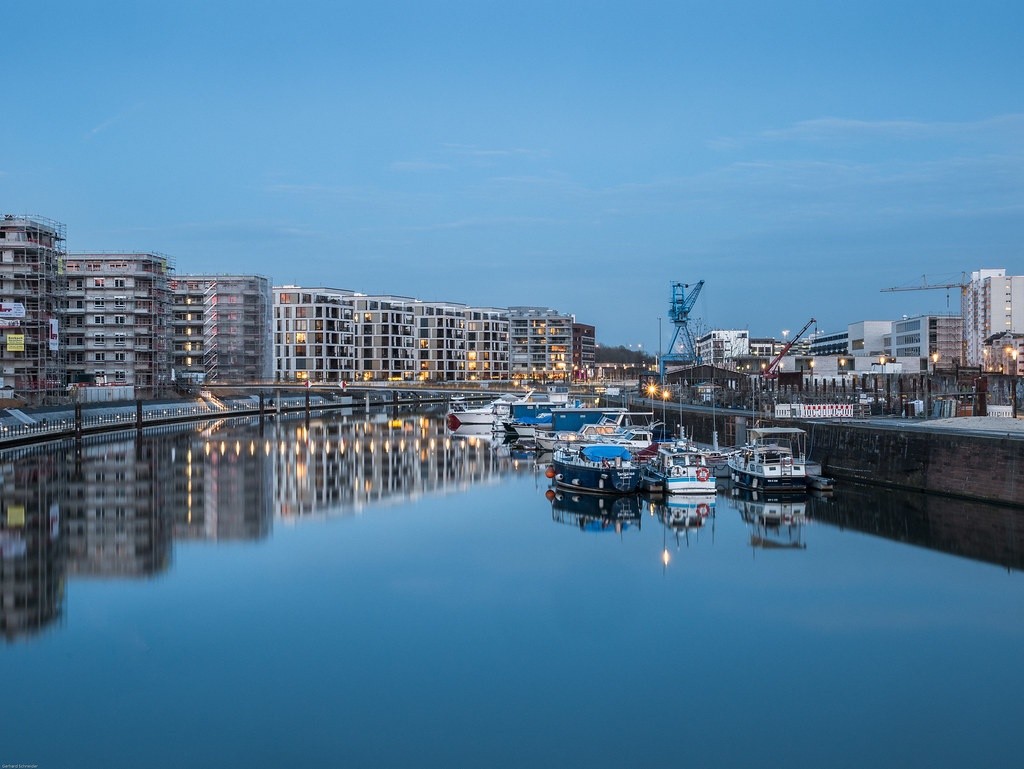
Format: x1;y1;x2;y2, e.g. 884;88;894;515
662;390;669;441
648;383;658;422
879;356;887;415
840;359;846;404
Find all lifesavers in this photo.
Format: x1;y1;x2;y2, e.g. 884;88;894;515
696;468;710;482
696;503;710;517
671;464;684;477
783;514;794;525
602;459;611;468
600;516;611;529
783;456;794;467
672;509;683;521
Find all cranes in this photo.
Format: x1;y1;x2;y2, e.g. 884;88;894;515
657;279;710;384
879;270;972;368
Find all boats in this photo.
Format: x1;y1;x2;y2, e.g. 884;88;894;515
727;493;810;552
643;441;721;495
727;425;807;494
549;488;645;537
659;493;720;537
440;384;655;497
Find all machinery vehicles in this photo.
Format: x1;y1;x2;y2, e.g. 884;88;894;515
761;316;819;379
